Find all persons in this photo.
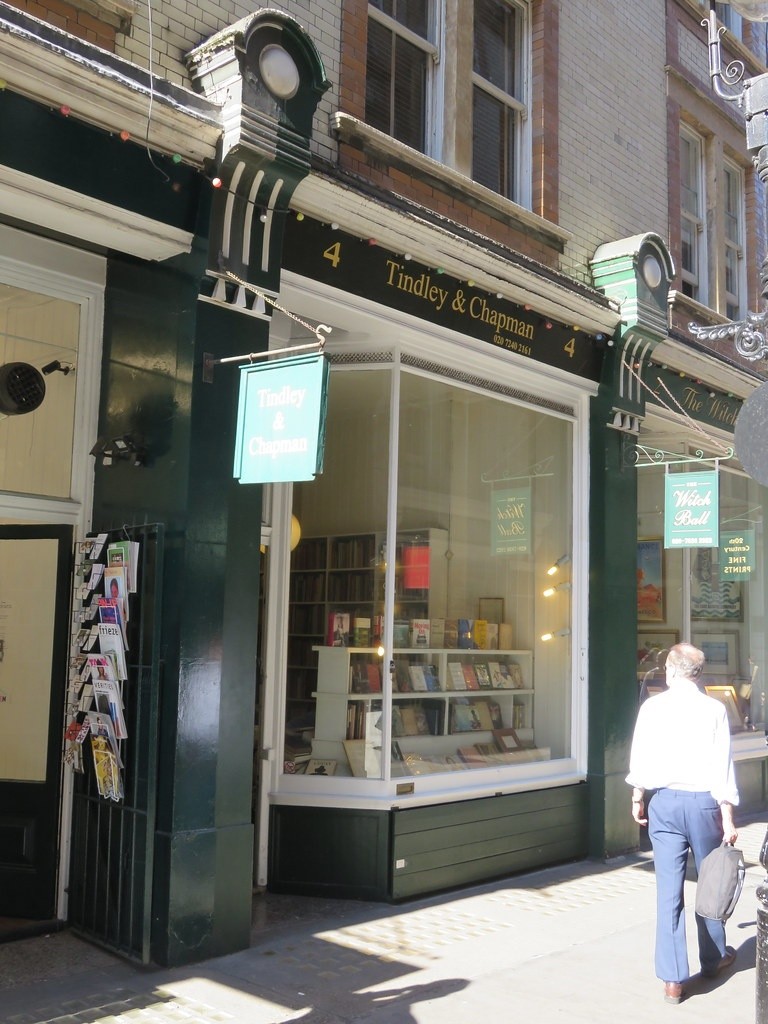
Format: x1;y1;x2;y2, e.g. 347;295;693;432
625;643;740;1004
639;649;669;704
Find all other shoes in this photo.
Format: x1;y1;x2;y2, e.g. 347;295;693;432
665;980;685;1004
700;946;736;976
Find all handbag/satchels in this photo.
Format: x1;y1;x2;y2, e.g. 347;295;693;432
696;840;746;921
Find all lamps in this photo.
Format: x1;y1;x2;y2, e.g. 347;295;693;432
113;436;148;468
541;630;569;641
544;582;571;596
547;552;570;576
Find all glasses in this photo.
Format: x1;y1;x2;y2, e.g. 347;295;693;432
663;664;673;671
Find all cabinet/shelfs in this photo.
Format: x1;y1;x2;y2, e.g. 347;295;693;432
311;644;535;769
255;527;449;731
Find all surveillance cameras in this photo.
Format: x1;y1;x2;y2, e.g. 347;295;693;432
42;360;60;376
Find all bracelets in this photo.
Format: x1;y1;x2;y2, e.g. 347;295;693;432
632;797;643;802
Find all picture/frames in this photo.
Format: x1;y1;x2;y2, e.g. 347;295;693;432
691;629;739;676
637;629;678;678
637;539;667;625
478;598;504;624
704;685;743;729
689;548;745;623
647;687;663;697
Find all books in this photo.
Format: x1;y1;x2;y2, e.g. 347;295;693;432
285;540;526;776
88;542;139;796
